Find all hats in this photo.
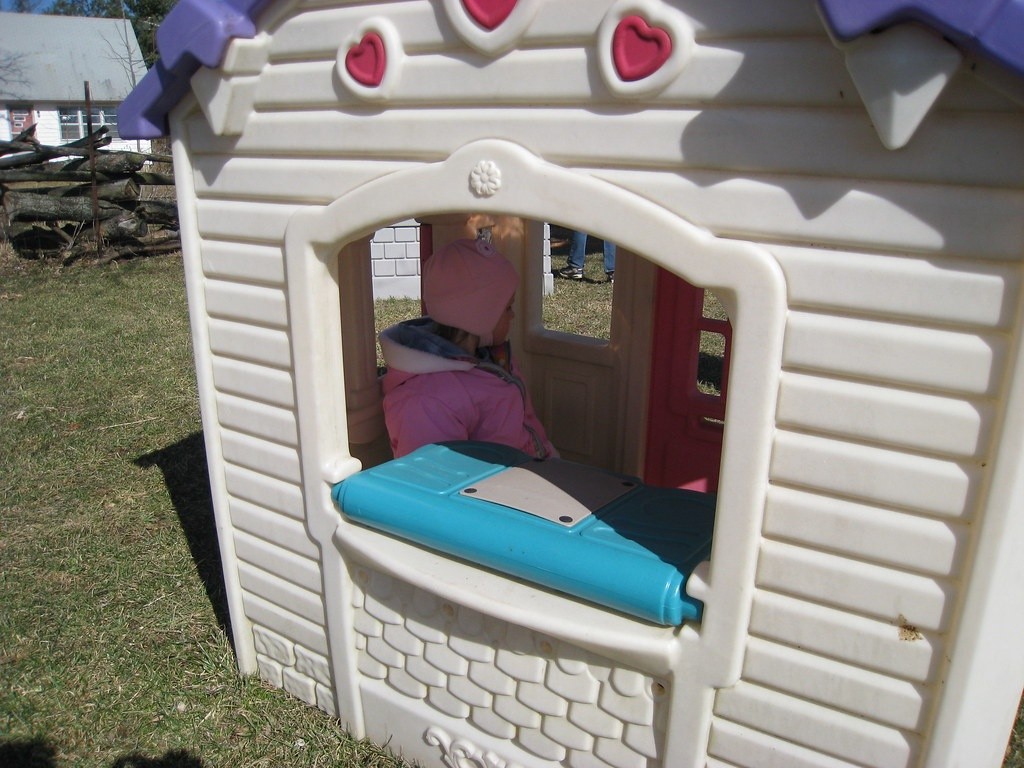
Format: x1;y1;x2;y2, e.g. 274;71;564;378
422;237;520;347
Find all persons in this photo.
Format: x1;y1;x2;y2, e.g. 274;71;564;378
378;236;560;462
557;229;617;283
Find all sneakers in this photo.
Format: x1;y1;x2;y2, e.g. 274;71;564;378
606;272;615;284
560;266;584;278
